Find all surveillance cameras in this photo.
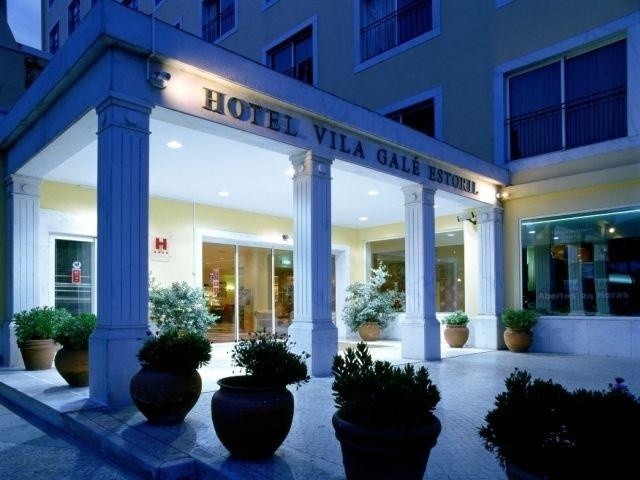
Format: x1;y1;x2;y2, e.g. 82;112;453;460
154;71;171;81
457;212;474;222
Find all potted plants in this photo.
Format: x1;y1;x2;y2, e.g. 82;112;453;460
441;305;473;350
477;365;640;480
332;341;441;480
341;257;405;341
213;324;314;460
499;305;541;351
44;311;99;388
127;324;216;426
11;303;75;371
